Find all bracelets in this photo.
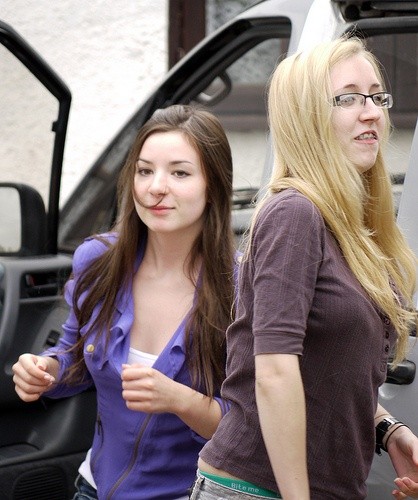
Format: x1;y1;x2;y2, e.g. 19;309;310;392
372;413;411;457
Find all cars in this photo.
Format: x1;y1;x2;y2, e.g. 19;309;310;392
1;1;418;500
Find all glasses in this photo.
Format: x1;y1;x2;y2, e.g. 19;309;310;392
330;92;393;110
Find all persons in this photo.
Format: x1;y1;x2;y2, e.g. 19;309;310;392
12;105;247;500
190;35;418;500
